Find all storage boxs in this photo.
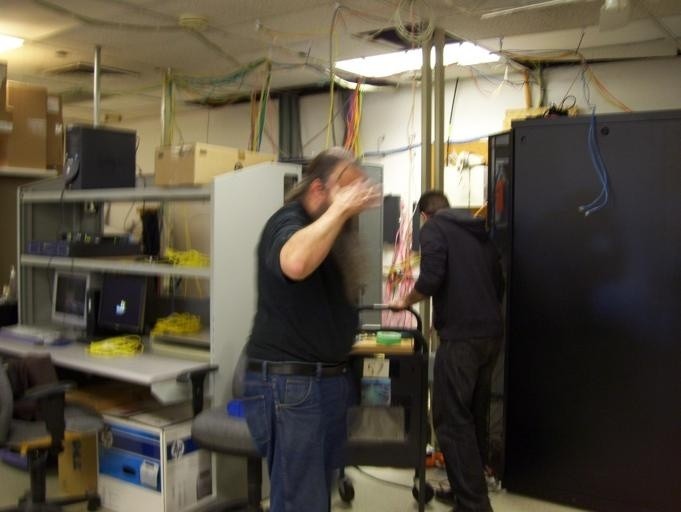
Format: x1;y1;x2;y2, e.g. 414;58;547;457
152;143;278;190
0;64;66;171
58;384;214;512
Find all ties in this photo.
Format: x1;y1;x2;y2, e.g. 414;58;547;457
435;486;462;512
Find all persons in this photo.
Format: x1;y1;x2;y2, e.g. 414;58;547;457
243;145;382;512
391;191;504;512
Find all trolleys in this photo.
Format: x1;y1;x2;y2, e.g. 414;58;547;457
335;296;437;505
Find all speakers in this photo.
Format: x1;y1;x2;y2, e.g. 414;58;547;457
140;207;161;256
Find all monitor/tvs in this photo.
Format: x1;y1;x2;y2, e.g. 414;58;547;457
50;269;95;330
95;272;158;335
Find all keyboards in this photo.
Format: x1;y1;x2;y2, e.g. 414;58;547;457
0;323;61;345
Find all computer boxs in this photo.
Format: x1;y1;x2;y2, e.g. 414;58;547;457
65;124;137;190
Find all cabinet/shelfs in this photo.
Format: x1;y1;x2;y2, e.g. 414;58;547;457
0;163;385;512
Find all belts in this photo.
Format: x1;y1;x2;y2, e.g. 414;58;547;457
248;363;349;375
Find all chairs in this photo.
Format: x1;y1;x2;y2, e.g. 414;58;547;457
0;352;102;512
191;341;269;512
